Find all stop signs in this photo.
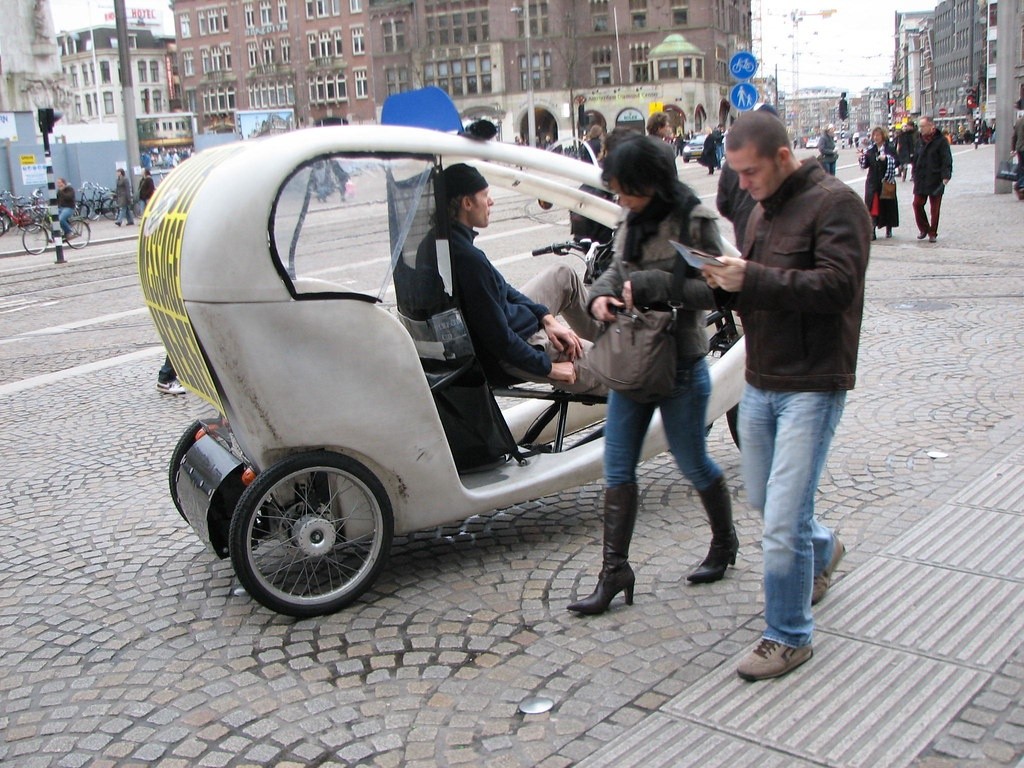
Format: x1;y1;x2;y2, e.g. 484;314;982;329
938;108;946;117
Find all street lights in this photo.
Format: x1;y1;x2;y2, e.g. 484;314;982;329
509;0;536;147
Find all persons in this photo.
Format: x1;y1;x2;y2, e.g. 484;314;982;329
56;178;76;237
1009;115;1024;201
567;131;739;615
701;111;873;680
514;124;605;163
416;164;607;397
794;136;808;149
847;132;873;147
717;104;779;251
858;126;902;240
569;125;642;270
115;168;135;226
818;124;839;176
891;121;921;182
942;118;996;145
663;123;725;175
156;353;185;394
141;145;191;170
308;161;349;202
646;112;670;138
910;115;953;242
138;169;154;215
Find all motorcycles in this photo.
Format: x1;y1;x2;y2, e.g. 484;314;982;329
133;122;748;619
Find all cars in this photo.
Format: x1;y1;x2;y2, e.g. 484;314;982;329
805;139;818;148
682;133;724;163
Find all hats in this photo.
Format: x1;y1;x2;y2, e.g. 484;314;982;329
827;124;833;130
589;125;602;138
433;164;489;208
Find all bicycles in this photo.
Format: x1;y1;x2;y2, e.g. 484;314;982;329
0;188;49;238
22;206;92;256
70;181;147;226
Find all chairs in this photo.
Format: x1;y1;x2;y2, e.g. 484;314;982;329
386;158;610;454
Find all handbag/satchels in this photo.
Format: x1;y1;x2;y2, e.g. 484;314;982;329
996;156;1019;181
133;189;140;202
587;304;678;404
880;180;895;199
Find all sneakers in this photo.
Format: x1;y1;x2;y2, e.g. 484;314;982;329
156;376;186;394
811;533;845;605
738;639;813;681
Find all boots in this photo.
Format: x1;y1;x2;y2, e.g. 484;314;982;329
567;483;638;613
687;472;739;583
886;226;892;237
871;227;876;240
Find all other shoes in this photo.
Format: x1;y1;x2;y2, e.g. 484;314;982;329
126;222;134;225
66;232;73;238
917;227;930;239
929;236;936;242
115;221;121;226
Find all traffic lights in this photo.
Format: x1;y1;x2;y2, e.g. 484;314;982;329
37;108;63;133
969;86;979;104
